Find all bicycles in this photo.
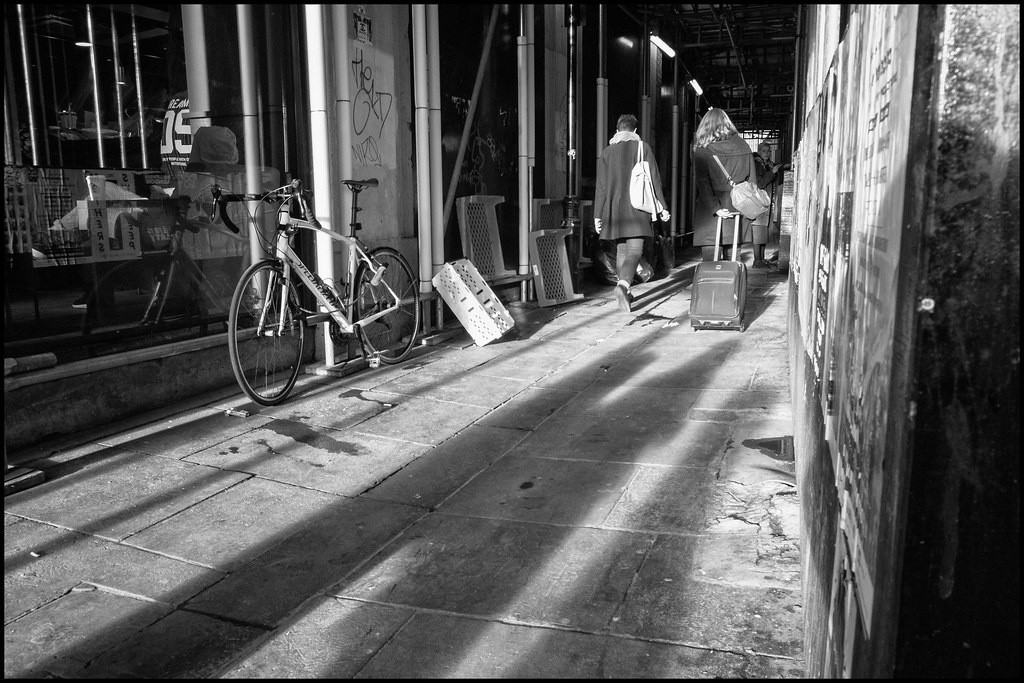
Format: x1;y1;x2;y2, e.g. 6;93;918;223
211;177;422;408
79;187;260;356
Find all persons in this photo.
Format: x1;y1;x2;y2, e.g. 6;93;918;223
692;108;758;262
752;142;791;267
594;114;670;312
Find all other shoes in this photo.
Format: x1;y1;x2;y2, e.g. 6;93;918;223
615;285;631;312
762;259;777;267
752;260;770;268
628;293;633;303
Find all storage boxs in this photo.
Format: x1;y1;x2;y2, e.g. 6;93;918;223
456;194;517;280
432;258;515;348
527;198;595;307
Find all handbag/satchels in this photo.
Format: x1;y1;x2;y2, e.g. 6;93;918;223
629;141;663;221
730;181;771;219
184;126;239;172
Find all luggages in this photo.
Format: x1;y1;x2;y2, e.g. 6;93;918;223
690;212;747;332
